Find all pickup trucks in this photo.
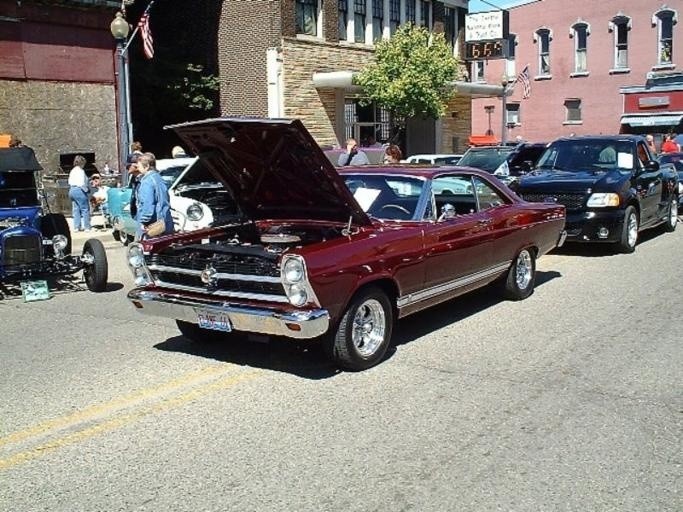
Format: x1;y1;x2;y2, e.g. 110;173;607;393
509;132;680;255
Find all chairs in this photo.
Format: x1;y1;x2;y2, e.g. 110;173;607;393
591;147;617;171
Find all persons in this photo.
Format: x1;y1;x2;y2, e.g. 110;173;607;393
125;141;174;242
381;143;402;164
172;146;190;158
645;135;656;155
660;133;680;154
338;139;371;166
67;156;109;233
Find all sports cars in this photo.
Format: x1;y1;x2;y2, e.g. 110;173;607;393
1;147;108;302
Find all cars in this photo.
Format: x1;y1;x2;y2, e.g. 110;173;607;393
653;151;682;216
105;152;244;249
453;138;553;187
124;113;569;376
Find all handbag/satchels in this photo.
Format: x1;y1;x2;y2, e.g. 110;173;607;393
145;219;166;237
